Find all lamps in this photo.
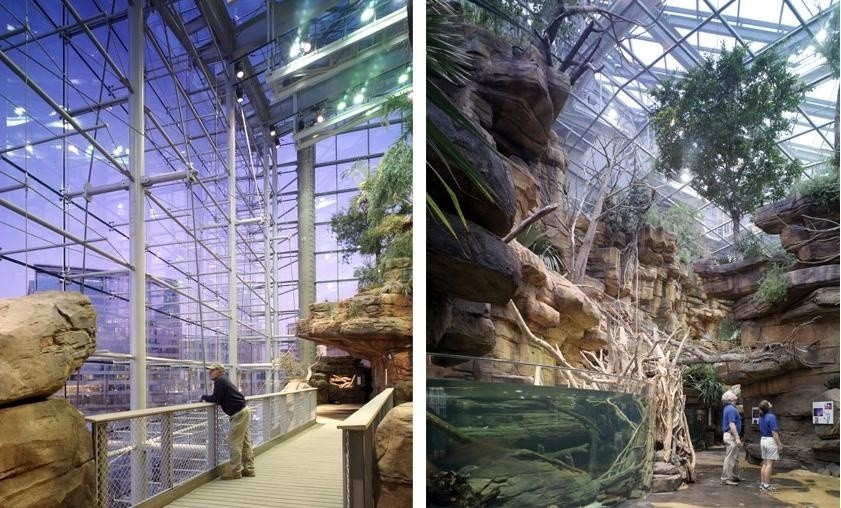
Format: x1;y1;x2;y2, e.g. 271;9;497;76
234;61;282;150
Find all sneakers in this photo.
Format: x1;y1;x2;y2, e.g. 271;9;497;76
721;477;746;486
222;473;242;479
760;483;774;491
241;469;255;476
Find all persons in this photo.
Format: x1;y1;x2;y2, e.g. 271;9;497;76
722;390;747;486
759;399;785;491
199;362;256;480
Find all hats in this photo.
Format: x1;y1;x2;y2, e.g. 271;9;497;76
206;362;226;372
